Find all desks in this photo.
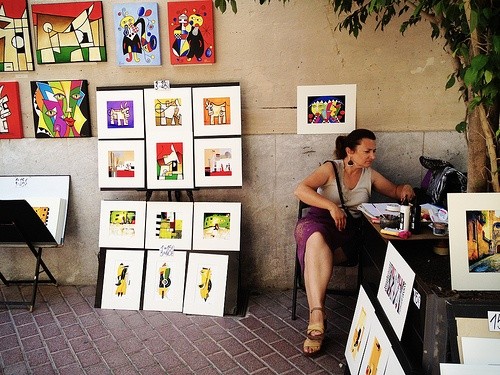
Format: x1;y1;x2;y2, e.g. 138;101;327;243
358;203;500;375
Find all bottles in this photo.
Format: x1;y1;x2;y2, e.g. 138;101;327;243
409;198;421;235
433;222;448;236
399;198;410;232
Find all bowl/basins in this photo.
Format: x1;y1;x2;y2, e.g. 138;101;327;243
421;221;432;232
380;214;399;229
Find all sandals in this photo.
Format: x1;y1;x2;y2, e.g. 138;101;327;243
302;307;328;355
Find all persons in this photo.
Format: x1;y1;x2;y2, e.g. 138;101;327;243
293;129;415;357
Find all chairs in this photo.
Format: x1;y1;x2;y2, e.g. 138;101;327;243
292;200;363;321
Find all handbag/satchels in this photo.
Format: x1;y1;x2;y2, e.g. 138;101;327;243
341;217;363;262
424;160;467;211
